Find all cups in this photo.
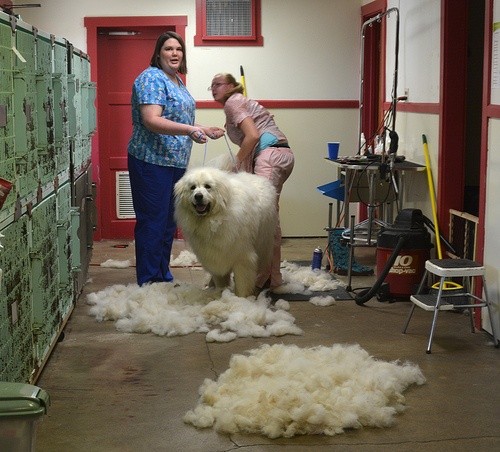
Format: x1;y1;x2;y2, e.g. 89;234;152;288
328;142;340;160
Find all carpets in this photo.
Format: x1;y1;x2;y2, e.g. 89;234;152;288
265;259;354;304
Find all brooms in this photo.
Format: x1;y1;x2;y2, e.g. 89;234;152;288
420;133;467;311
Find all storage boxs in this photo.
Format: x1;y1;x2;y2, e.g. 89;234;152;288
328;229;375;277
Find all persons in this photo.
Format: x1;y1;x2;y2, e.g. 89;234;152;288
211;72;295;299
126;31;226;288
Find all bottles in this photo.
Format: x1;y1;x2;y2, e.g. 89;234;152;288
312;245;322;271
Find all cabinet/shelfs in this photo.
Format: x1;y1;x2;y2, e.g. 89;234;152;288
0;8;99;390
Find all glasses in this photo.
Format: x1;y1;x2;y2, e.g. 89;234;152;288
207;82;232;91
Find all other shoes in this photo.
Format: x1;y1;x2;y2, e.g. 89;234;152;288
269;272;283;290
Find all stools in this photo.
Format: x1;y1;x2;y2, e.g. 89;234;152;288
401;257;499;356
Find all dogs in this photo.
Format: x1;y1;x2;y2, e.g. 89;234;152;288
173;165;279;300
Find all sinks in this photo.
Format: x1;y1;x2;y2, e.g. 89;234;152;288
326;154;427;203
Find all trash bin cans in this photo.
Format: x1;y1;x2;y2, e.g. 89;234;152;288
0;381;52;451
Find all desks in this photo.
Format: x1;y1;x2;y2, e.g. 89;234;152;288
321;157;428;293
